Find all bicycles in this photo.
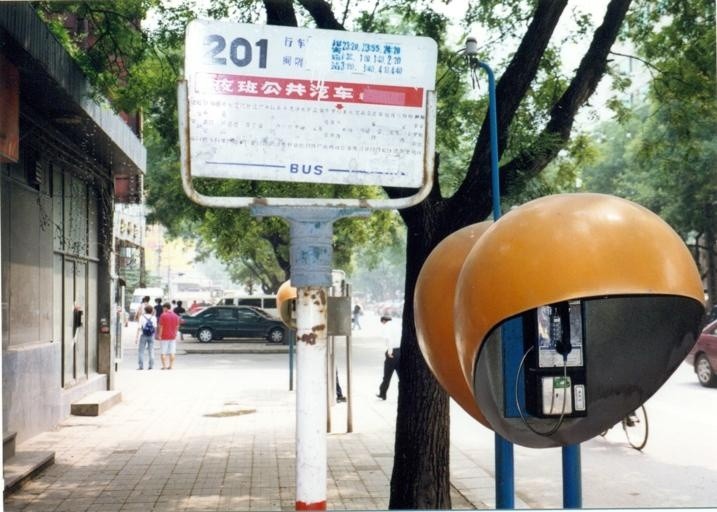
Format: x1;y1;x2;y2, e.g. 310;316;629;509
600;404;649;450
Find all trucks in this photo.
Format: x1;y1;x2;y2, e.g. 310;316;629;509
130;287;163;319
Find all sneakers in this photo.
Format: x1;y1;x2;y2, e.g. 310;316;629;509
137;366;171;370
377;394;386;399
337;397;346;402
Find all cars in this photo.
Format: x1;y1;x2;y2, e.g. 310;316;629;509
179;305;288;343
123;309;130;327
683;319;717;386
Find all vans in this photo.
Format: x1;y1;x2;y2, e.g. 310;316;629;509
213;294;279;320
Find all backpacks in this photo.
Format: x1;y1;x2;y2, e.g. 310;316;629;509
142;314;154;336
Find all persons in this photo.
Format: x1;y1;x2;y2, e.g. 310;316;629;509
132;295;205;341
157;303;180;371
374;314;403;401
136;304;158;371
351;304;363;331
